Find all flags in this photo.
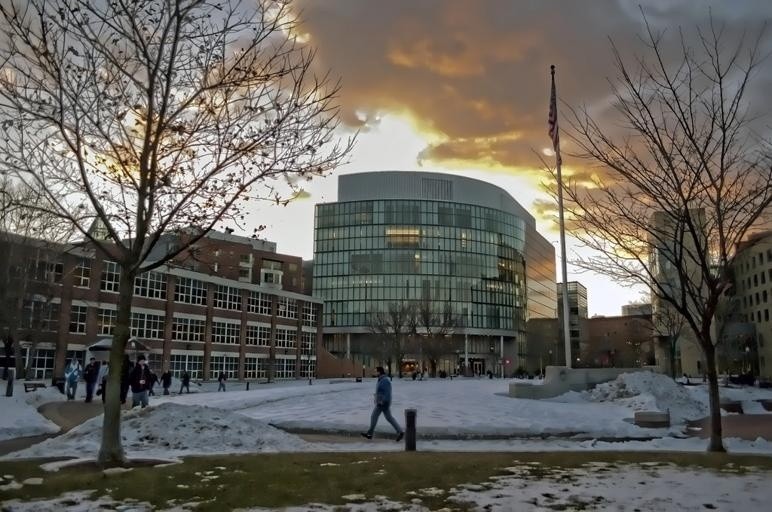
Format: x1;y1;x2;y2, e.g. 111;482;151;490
547;72;562;163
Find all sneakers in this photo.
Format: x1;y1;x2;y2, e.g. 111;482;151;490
361;433;372;439
396;432;404;441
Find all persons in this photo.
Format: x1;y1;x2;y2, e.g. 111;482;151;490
130;355;152;409
217;371;227;392
148;369;160;397
360;366;405;442
63;356;82;401
121;354;133;404
84;357;99;403
98;359;110;402
159;368;171;395
178;369;190;395
